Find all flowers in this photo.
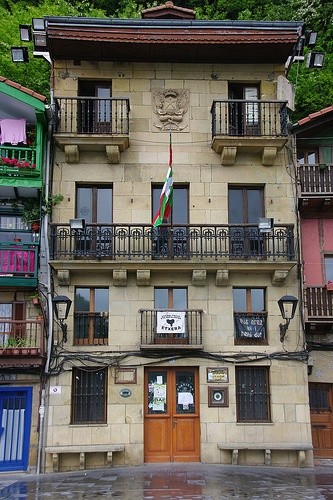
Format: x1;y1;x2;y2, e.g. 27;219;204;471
328;280;332;284
2;157;36;169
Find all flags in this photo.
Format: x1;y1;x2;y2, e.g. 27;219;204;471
152;133;174;226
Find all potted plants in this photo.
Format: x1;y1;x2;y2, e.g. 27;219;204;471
21;194;64;230
26;293;47;320
0;336;37;354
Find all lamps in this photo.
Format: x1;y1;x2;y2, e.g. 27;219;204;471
51;296;72;343
258;218;274;233
11;18;48;63
278;296;299;342
70;219;85;229
286;23;325;78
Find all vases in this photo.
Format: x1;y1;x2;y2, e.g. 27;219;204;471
14;239;21;242
325;285;333;290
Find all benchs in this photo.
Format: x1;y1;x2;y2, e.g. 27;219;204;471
218;443;314;467
45;445;125;473
